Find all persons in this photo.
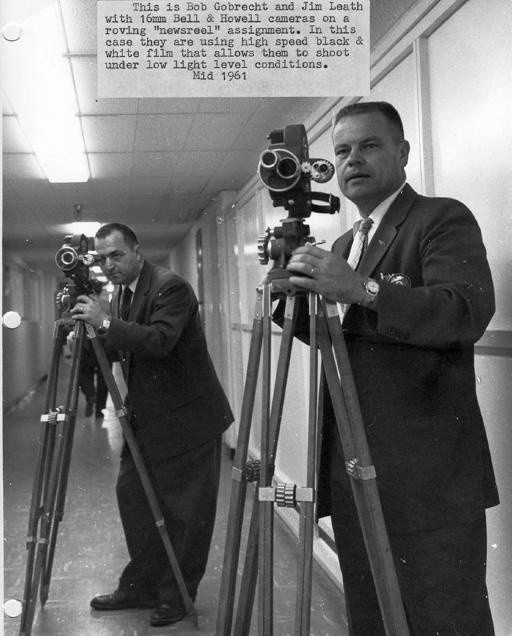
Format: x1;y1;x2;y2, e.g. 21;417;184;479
81;277;121;419
272;98;501;636
70;220;236;628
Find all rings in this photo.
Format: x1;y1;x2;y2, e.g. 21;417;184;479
80;307;83;312
308;265;317;277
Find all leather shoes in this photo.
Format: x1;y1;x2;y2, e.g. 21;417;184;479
91;586;161;610
85;401;93;417
96;412;104;418
149;602;185;626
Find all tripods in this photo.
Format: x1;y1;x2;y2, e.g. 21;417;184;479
215;220;411;636
19;295;197;636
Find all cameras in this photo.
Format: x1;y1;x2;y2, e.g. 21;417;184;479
54;233;101;283
257;123;335;218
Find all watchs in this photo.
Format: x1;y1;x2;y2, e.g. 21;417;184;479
356;276;380;308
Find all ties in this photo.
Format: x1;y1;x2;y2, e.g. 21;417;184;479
120;286;132;322
337;219;374;312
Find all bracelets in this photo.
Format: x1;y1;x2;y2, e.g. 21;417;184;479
96;314;111;336
